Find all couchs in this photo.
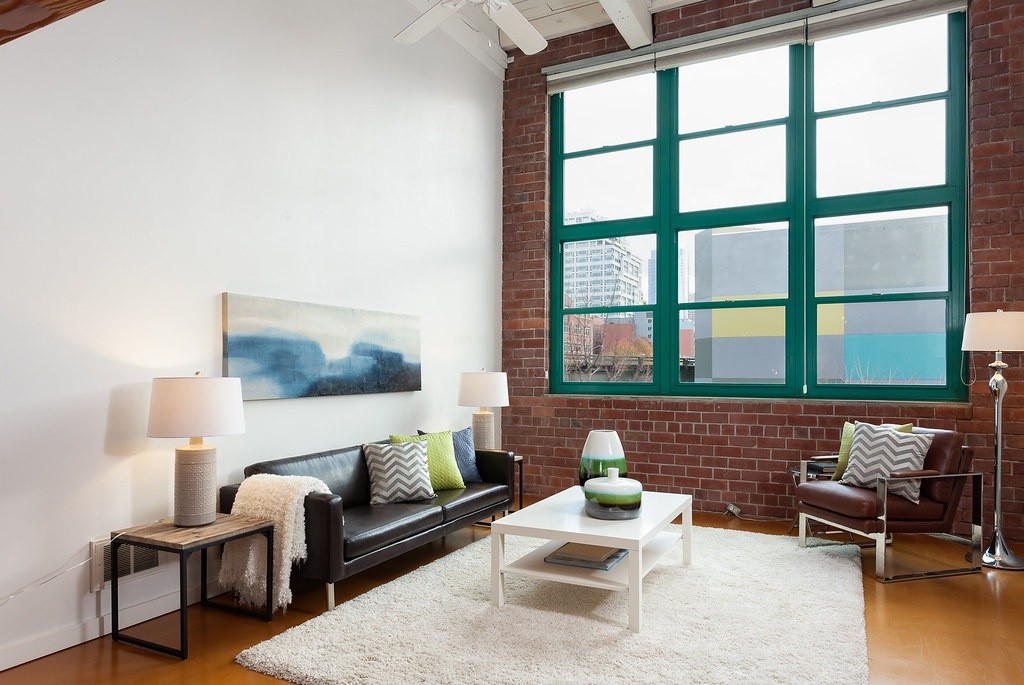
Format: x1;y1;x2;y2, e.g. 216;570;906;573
797;424;983;584
220;437;515;613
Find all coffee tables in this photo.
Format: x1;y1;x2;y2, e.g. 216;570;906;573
492;486;695;632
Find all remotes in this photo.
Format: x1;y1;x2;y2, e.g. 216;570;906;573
810;455;839;461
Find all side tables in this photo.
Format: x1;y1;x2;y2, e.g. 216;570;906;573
472;456;526;525
107;512;275;660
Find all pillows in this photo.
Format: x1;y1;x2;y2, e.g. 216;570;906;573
838;421;934;504
363;440;438;504
390;430;466;491
830;422;914;481
417;425;483;485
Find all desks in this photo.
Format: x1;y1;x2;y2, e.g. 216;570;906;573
789;468;855;541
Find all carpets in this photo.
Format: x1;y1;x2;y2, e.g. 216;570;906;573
237;520;875;684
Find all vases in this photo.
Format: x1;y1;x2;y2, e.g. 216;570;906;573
579;429;629;499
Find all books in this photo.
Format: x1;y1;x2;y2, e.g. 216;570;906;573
808;463;837;472
544;542;628;570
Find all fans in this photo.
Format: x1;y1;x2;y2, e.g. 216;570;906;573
393;0;549;55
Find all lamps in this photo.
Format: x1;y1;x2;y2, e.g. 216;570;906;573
456;367;510;449
960;309;1024;573
146;371;246;528
